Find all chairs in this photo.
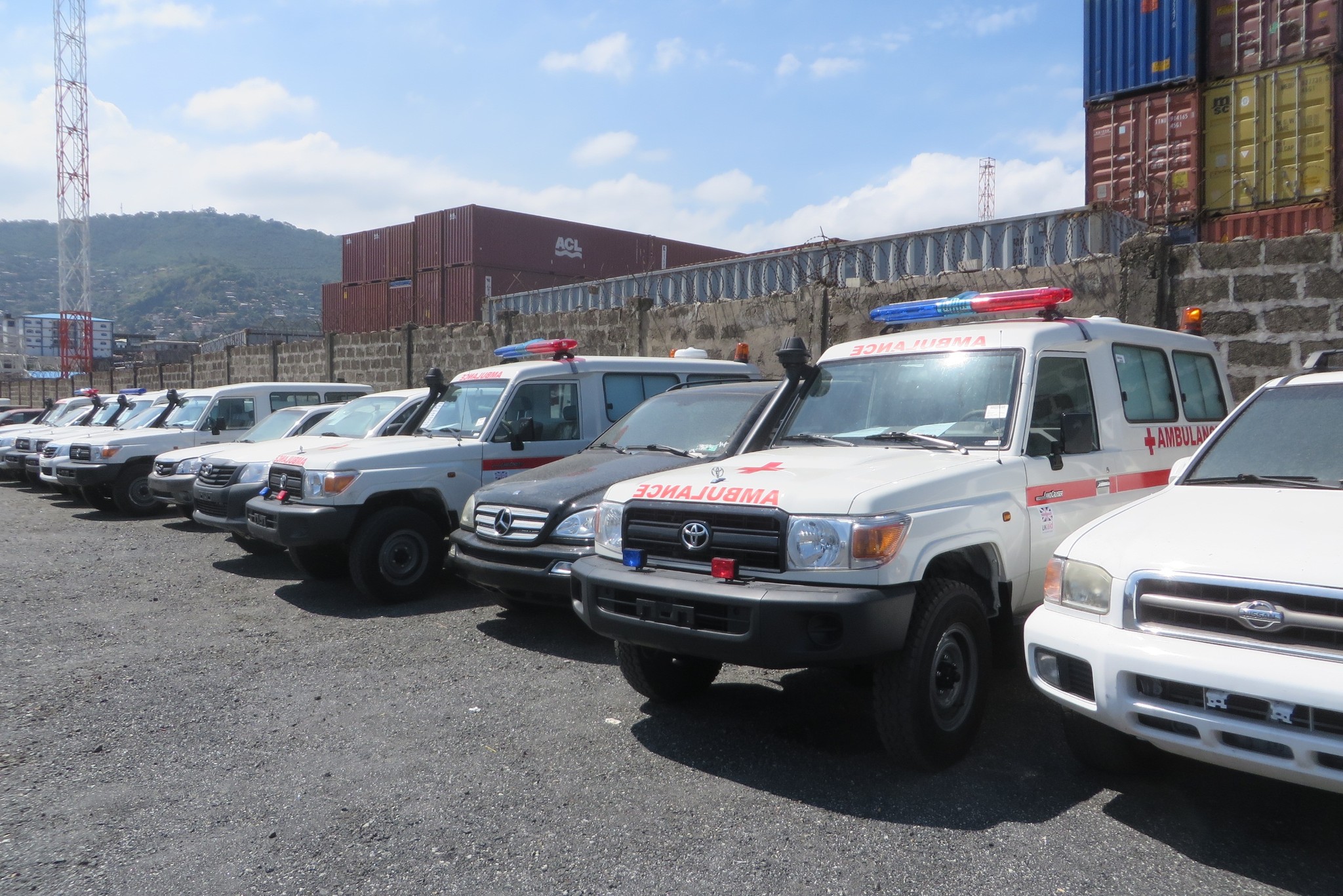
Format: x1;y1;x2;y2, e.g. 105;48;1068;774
506;395;539;442
1031;390;1085;457
226;405;248;428
560;405;581;440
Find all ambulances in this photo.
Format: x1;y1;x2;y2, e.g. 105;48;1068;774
245;337;762;609
570;289;1235;760
0;381;376;513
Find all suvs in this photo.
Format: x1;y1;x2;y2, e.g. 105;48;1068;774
1022;350;1343;797
445;376;868;612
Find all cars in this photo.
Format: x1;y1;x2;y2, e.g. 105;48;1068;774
146;400;396;520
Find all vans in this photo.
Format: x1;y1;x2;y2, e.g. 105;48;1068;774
193;388;566;537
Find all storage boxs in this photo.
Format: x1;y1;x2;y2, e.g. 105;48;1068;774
320;1;1342;334
